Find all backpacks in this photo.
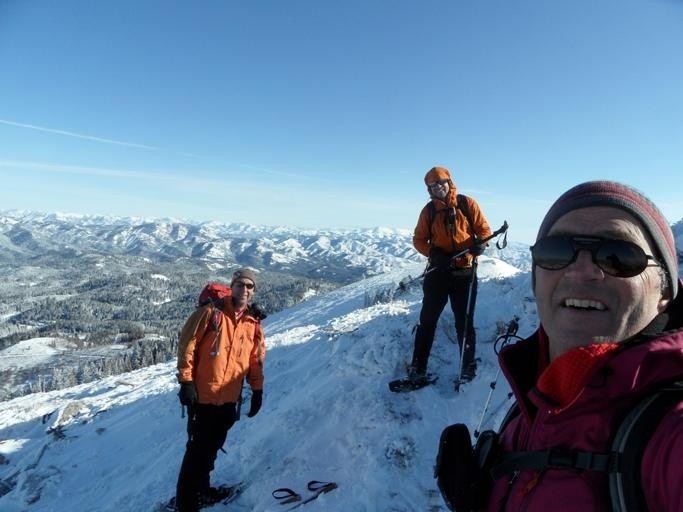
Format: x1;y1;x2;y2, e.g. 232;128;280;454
199;283;232;307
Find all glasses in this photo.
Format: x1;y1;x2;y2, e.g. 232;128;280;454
233;280;256;289
529;236;665;278
428;178;449;188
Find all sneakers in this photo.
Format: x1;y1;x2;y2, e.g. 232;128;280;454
459;362;477;384
402;364;427;385
176;487;220;512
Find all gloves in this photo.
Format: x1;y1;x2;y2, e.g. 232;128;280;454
468;238;486;258
247;390;264;418
429;246;451;268
178;381;198;408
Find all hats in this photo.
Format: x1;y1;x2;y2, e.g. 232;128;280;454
231;269;257;294
530;180;679;301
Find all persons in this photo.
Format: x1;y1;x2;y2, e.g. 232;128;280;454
387;165;491;393
434;178;682;512
171;267;269;512
